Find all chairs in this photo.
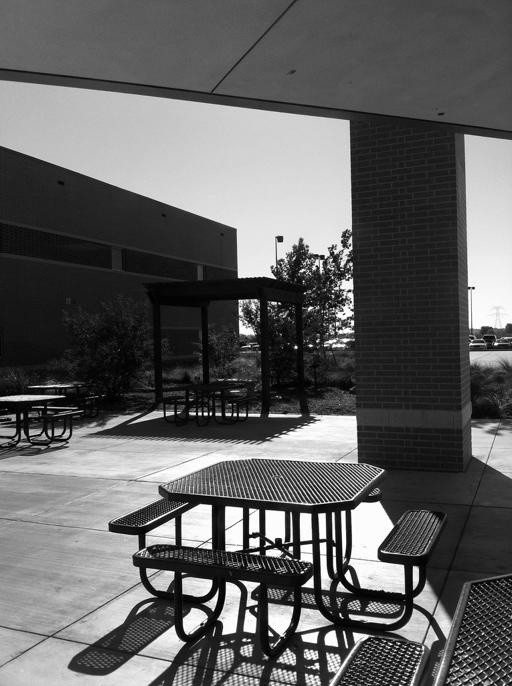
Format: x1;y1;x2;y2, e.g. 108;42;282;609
1;379;255;451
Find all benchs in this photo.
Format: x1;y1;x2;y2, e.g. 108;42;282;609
377;505;447;566
132;543;316;590
326;636;428;686
368;487;381;500
108;497;194;534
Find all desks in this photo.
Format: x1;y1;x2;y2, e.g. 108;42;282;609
434;575;510;685
138;458;426;660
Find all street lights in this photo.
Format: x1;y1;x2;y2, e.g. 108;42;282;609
468;286;475;331
274;235;284;269
317;254;325;270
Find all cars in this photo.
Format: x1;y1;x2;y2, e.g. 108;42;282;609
468;332;512;350
240;338;355;350
